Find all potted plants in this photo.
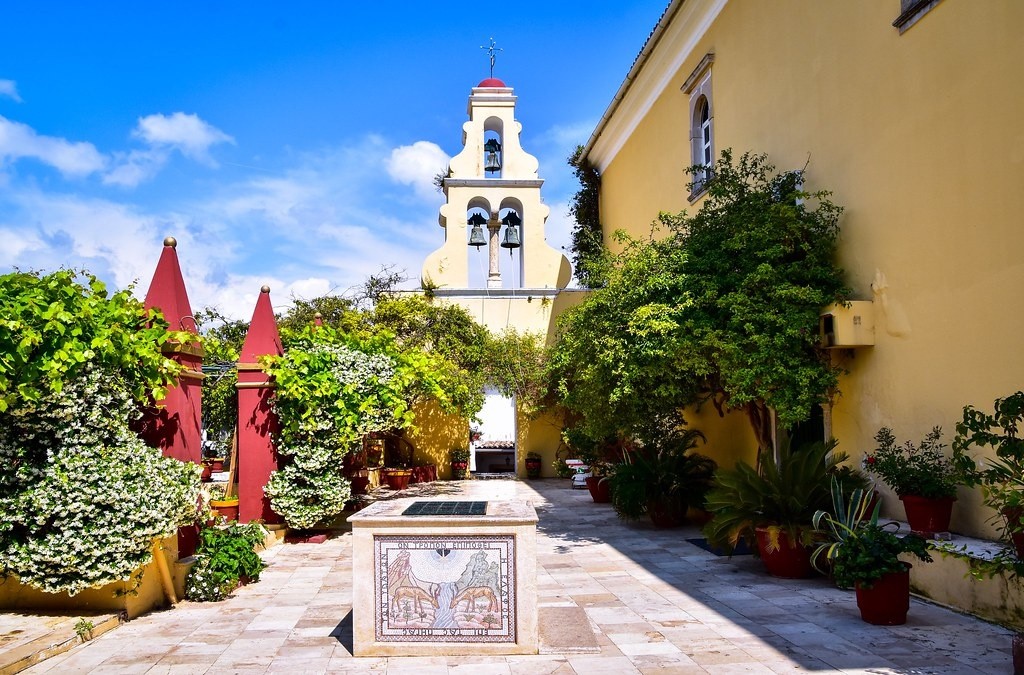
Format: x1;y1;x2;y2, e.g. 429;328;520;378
808;473;933;625
524;451;542;481
943;391;1024;560
930;541;1024;675
208;484;239;522
599;428;718;526
201;454;226;481
861;427;964;538
704;433;862;578
470;426;483;442
449;449;471;480
562;422;613;504
386;468;412;490
